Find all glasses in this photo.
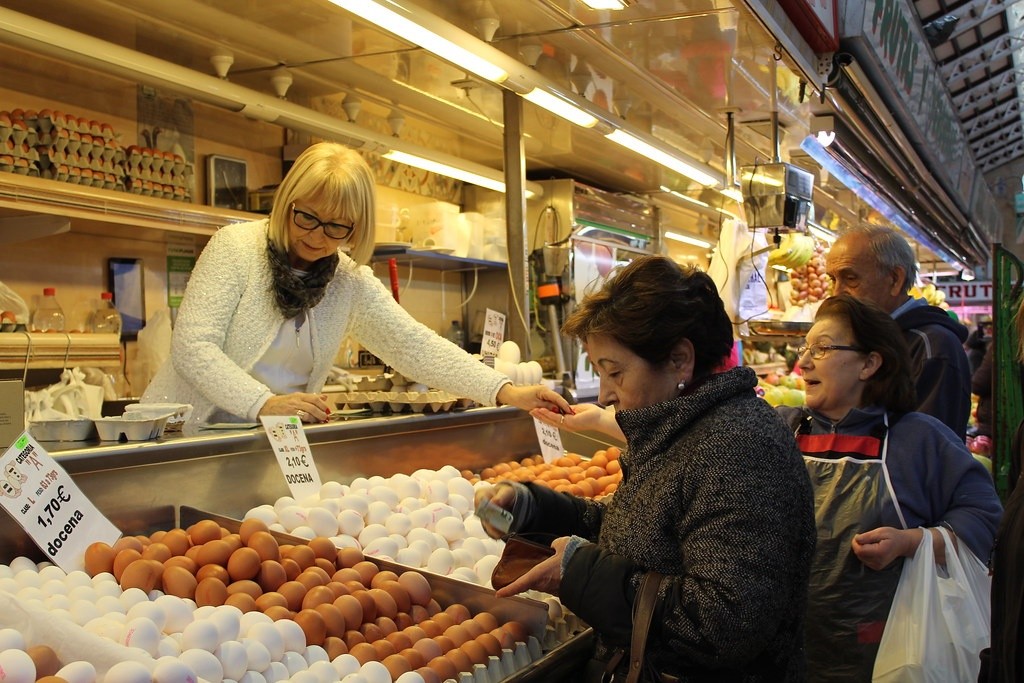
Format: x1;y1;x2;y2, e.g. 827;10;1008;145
797;340;858;359
290;205;354;239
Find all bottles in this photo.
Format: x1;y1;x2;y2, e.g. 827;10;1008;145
447;320;465;349
92;292;123;391
33;287;66;333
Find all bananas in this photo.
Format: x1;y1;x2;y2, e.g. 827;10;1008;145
768;232;813;270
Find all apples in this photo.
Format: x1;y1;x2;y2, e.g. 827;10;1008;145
754;374;807;407
964;433;993;459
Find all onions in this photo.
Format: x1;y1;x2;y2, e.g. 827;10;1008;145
789;246;831;306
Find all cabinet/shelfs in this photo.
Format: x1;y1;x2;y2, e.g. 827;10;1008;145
1;3;752;400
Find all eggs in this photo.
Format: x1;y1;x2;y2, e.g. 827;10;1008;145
0;108;187;196
0;519;529;683
0;311;90;333
242;445;623;622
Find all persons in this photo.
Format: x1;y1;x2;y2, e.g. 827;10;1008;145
775;292;1007;683
472;256;816;683
966;300;1024;436
826;225;970;444
140;142;576;416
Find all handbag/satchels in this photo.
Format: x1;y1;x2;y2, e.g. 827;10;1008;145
872;525;991;682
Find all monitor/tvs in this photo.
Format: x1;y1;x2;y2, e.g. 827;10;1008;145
109;257;146;331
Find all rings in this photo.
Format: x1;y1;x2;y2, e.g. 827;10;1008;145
561;417;564;423
297;410;303;419
